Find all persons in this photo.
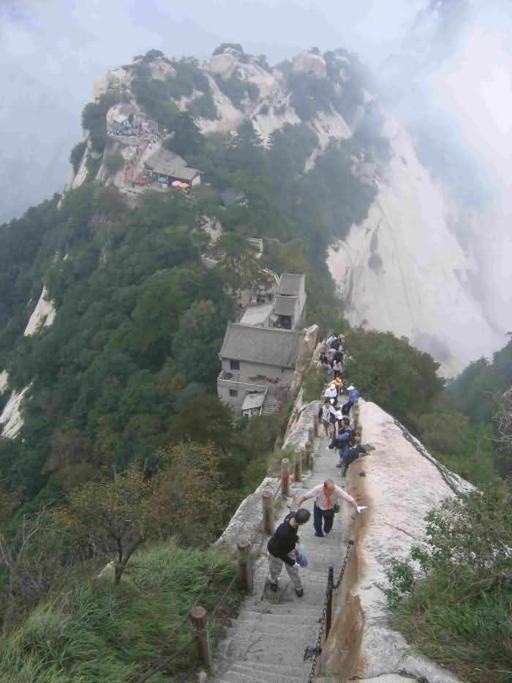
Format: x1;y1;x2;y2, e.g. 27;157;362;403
268;508;308;596
316;330;370;477
296;478;368;537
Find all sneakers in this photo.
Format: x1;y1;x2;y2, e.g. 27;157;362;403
296;588;302;596
272;584;277;591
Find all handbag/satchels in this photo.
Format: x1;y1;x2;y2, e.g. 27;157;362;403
333;504;340;513
